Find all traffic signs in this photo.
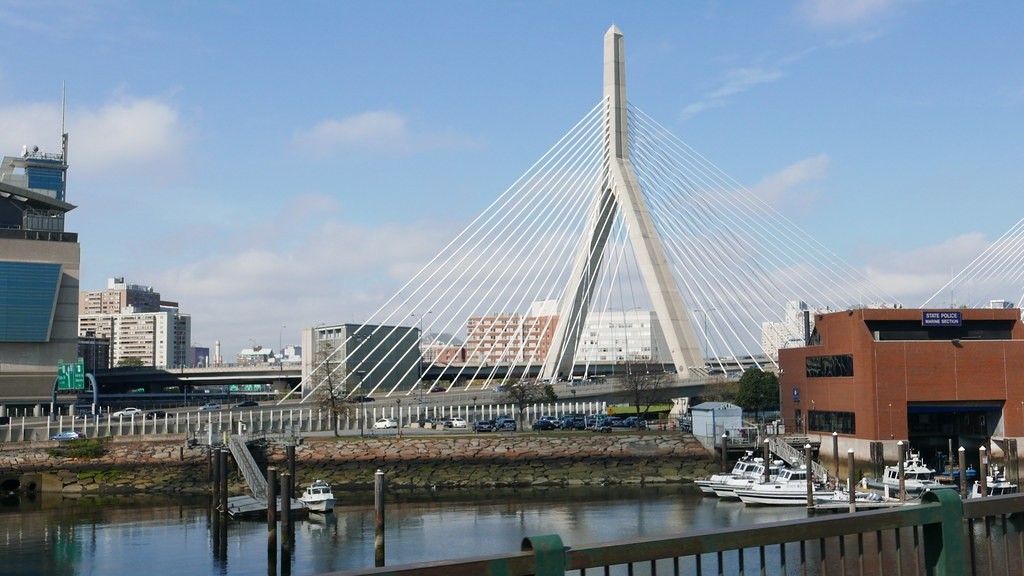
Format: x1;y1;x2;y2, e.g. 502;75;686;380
57;357;85;390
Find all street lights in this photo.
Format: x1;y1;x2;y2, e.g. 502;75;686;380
570;389;576;421
396;398;401;435
694;309;717;365
472;396;477;433
609;306;642;377
411;311;432;399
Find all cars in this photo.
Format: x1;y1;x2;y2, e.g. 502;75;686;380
74;411;104;420
113;407;142;417
199;402;221;410
472;414;646;431
431;387;447;392
349;396;375;403
147;410;173;419
374;418;466;429
493;377;607;392
50;431;86;440
234;401;258;408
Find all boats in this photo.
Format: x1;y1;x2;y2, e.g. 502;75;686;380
694;447;881;506
971;464;1018;499
862;451;959;498
297;480;337;511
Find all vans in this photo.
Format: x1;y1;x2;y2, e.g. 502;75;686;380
328;390;347;399
